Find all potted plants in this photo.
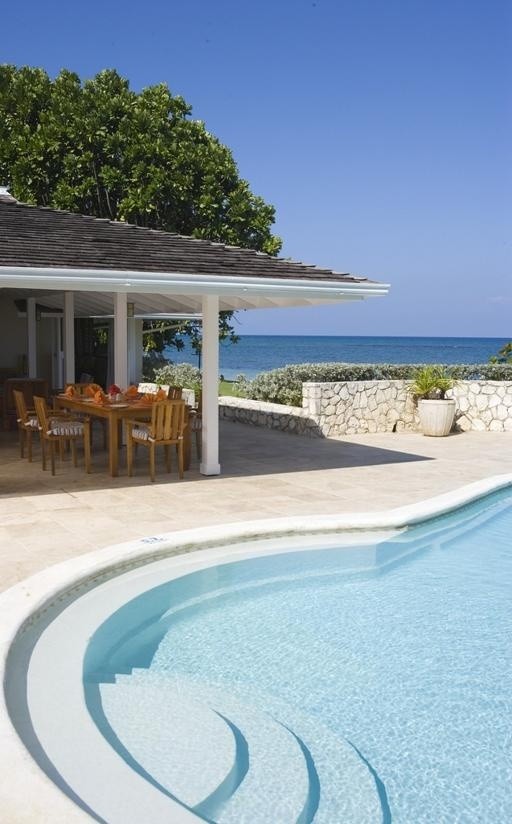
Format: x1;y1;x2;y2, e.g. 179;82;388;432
407;363;461;438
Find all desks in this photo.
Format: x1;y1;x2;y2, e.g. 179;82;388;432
53;393;191;477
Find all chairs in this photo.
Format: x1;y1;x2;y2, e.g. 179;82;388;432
126;400;185;482
65;383;91;396
13;390;64;463
183;391;202;471
33;396;84;475
166;386;182;399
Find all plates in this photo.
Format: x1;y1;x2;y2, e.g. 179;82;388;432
109;404;130;409
56;393;69;401
83;398;102;404
